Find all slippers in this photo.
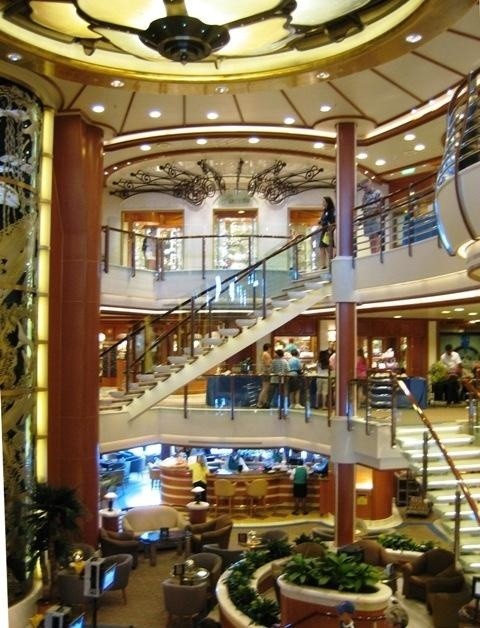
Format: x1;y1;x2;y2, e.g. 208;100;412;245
322;408;328;410
314;406;318;409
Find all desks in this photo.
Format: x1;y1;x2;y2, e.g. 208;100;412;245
205;374;429;409
430;377;480;407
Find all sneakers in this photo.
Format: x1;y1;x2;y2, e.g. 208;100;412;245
303;511;309;515
292;511;299;515
256;405;270;409
289;403;294;409
293;403;305;409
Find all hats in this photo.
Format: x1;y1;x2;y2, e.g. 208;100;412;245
335;601;355;614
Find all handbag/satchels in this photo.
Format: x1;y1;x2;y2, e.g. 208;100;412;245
321;231;330;246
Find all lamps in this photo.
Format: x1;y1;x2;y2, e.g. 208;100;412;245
0;0;425;81
104;492;117;511
190;486;205;504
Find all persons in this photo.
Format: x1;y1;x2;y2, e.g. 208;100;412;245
240;354;254;372
472;361;480;387
360;179;386;255
394;368;401;377
309;220;323;268
441;344;465;400
333;600;358;628
319;196;336;268
228;449;240;470
256;337;370;410
234;449;250;473
399;368;409;377
190;454;213;503
145;228;161;272
290;459;310;515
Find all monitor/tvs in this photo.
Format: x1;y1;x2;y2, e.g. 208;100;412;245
102;563;115;592
68;612;86;628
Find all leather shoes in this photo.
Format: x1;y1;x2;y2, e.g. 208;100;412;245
445;403;458;409
322;266;327;270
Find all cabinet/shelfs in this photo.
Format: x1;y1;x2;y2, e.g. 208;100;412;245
394;471;418;506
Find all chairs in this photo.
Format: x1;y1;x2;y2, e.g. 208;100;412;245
99;451;161;488
404;495;433;519
401;547;456;601
421;573;472;628
244;479;268;518
214;481;237;517
